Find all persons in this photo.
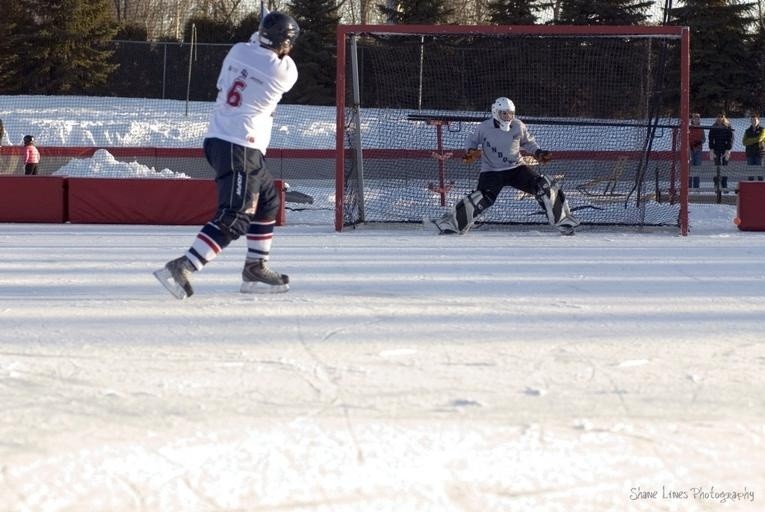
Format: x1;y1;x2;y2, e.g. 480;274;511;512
151;10;300;299
707;108;735;195
0;119;13;146
23;134;42;175
685;111;707;190
422;95;580;236
742;112;765;182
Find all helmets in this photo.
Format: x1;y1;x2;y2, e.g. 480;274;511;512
258;11;300;49
491;97;515;131
24;136;33;144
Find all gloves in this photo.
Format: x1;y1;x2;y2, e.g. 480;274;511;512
723;150;731;161
535;149;552;161
463;149;481;163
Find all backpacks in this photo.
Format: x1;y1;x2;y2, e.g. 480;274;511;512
710;149;716;160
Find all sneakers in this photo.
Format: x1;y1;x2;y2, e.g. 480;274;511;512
242;259;288;285
560;229;573;235
166;256;195;296
